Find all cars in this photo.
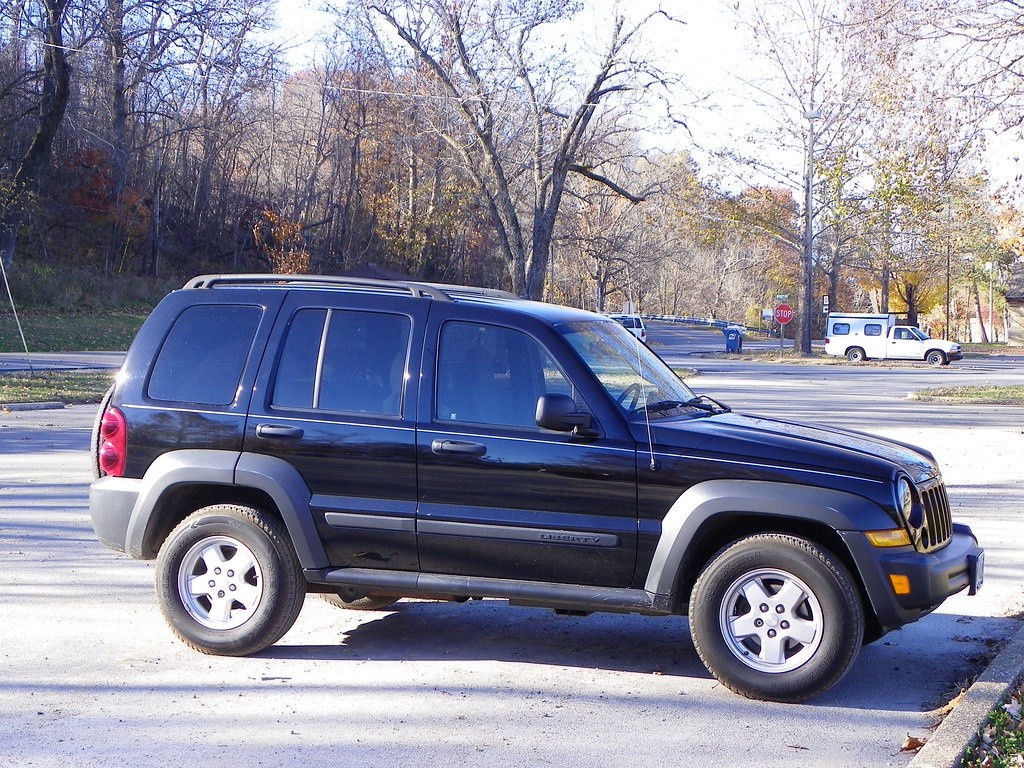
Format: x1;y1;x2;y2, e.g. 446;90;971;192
605;310;648;347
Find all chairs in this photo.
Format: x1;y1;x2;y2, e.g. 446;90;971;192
464;346;493;386
383;350;450;418
623;321;629;325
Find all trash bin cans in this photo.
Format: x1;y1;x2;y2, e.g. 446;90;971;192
721;325;747;353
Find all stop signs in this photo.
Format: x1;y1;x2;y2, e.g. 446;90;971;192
774;303;794;324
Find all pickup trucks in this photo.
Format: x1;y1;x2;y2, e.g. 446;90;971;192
824;311;963;367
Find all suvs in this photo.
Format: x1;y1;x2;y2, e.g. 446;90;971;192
90;272;985;705
